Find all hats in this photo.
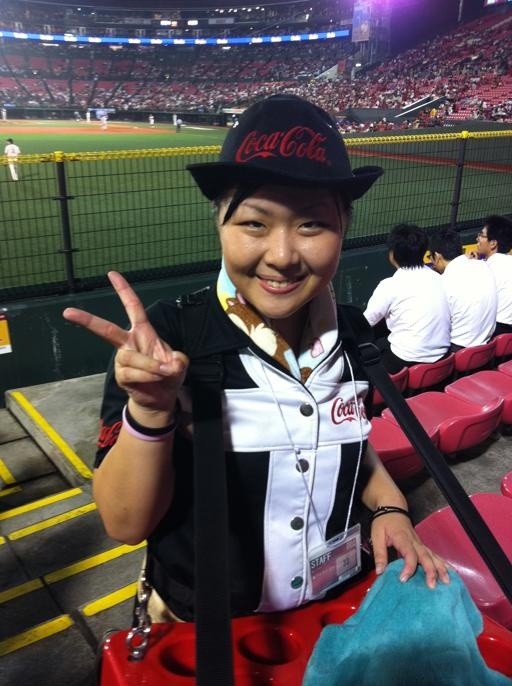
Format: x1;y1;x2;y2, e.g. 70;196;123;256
186;94;385;202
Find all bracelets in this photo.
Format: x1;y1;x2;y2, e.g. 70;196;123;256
368;505;413;523
122;404;174;442
125;407;176;436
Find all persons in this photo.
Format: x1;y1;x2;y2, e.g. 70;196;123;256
4;139;21;181
0;1;512;134
469;216;512;336
362;224;452;375
62;94;459;623
426;229;497;354
